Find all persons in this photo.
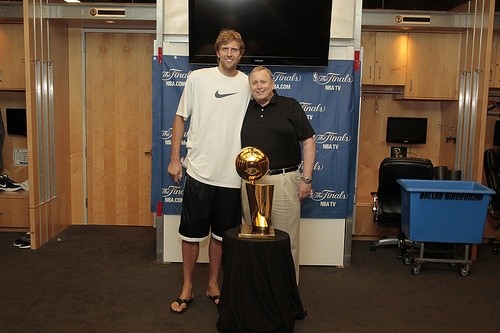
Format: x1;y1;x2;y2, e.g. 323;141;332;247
167;31;253;315
241;66;316;286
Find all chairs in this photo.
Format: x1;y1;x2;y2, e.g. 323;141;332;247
368;158;436;259
483;148;500;254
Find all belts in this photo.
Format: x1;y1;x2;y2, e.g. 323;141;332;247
268;167;298;176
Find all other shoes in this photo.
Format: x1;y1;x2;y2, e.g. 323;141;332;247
0;174;24;192
13;232;31;249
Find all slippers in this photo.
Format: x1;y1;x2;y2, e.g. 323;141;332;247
170;295;194;314
205;293;220;306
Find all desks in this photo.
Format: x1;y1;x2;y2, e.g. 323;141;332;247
215;228;307;333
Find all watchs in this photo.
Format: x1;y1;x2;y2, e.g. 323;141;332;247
301;177;312;183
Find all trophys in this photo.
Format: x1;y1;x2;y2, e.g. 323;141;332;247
236;147;275;240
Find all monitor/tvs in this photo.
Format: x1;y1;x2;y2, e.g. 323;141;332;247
6;107;27;136
187;0;333;69
385;117;428;144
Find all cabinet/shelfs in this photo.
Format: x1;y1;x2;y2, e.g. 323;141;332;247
361;31;460;101
